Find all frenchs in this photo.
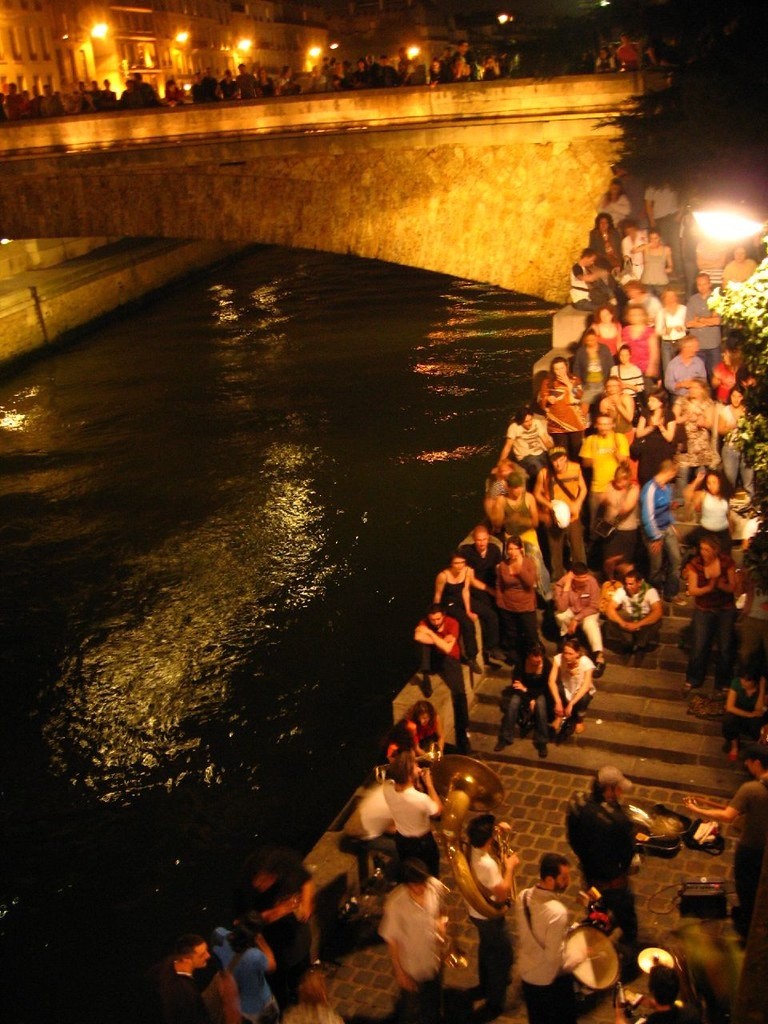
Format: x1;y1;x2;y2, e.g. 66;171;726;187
433;754;516;919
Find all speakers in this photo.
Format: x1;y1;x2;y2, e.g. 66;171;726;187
679;876;728;920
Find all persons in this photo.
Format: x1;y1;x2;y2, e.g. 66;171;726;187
0;31;706;121
159;160;768;1024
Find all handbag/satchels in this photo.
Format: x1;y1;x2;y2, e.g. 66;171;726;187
630;438;645;457
593;519;616;537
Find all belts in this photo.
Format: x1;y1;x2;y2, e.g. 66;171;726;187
663;340;678;344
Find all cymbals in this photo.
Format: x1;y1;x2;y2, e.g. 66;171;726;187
637;947;675;975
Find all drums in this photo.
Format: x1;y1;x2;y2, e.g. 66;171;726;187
561;924;621;995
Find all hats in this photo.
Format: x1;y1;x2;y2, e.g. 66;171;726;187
597;765;632;792
549;446;566;455
508;472;523;488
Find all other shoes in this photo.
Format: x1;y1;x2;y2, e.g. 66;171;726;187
422;678;433;698
575;723;584;734
592;661;606;678
456;740;471;753
728;746;738;762
469;660;482;674
681;681;692;693
490;650;506;661
537;743;547;758
622;651;644;667
495;740;512;751
